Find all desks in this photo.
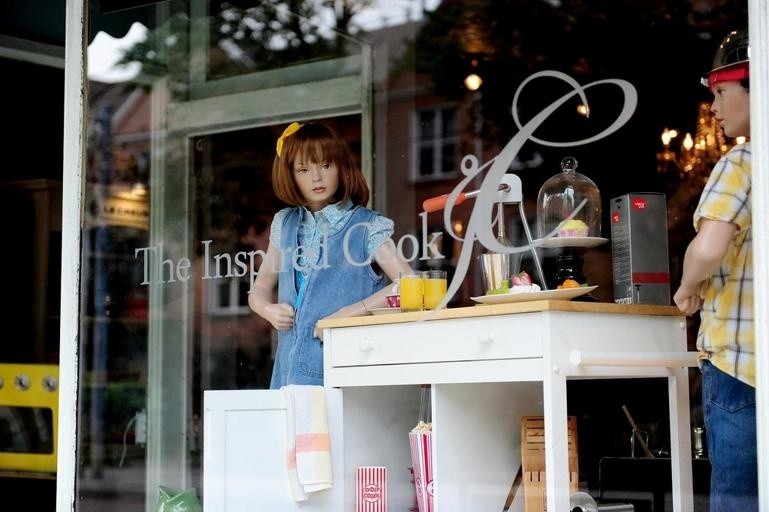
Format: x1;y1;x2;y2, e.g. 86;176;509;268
202;299;694;511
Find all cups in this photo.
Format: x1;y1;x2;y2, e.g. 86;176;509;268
398;269;424;313
424;270;449;311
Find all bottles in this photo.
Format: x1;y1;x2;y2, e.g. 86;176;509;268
419;384;431;425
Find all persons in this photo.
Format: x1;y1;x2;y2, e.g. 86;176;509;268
247;120;419;390
673;28;758;512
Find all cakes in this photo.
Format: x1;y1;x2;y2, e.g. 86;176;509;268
510;271;540;292
556;219;589;237
558;278;580;289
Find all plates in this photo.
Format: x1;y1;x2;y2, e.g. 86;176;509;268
366;307;402;316
531;236;610;250
469;285;599;305
